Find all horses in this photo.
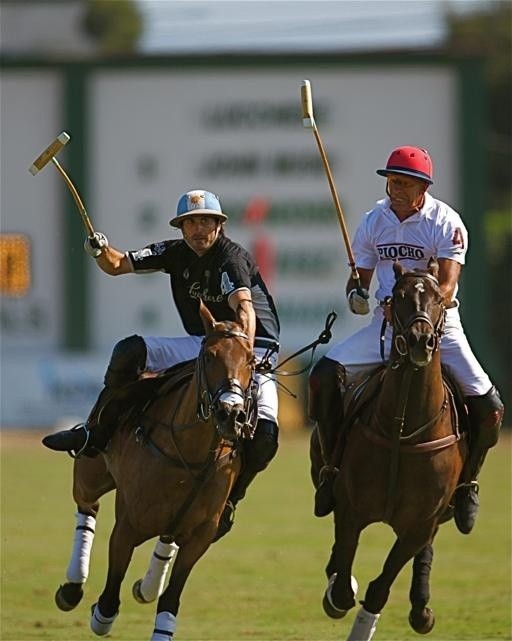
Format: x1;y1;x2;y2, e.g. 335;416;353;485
55;296;256;641
309;255;471;641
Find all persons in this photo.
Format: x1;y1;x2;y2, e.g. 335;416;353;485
33;189;282;538
308;145;505;535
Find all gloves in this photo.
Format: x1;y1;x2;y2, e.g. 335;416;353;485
83;232;109;257
347;287;370;315
382;295;392;324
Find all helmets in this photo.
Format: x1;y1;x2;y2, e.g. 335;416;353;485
376;146;434;184
170;190;228;228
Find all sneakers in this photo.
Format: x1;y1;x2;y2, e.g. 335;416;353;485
452;487;479;536
213;505;232;542
314;464;333;516
42;428;92;451
